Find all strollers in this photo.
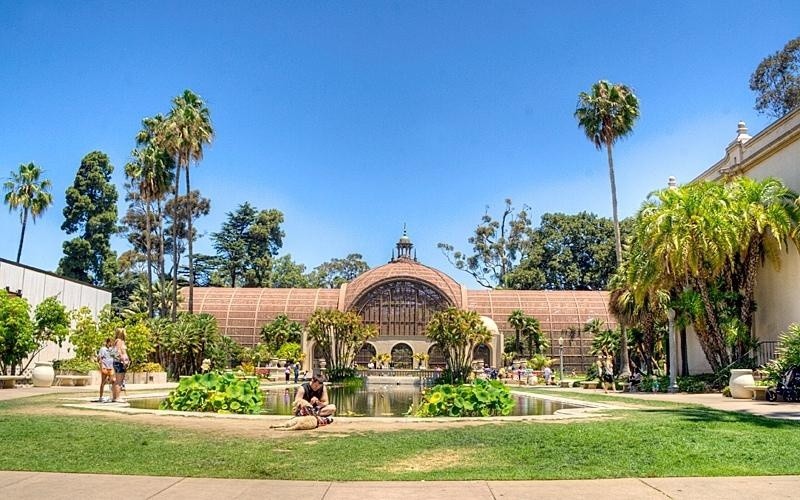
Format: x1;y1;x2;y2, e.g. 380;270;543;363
764;363;800;403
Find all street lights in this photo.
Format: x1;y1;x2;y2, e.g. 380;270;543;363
665;306;682;390
557;337;565;381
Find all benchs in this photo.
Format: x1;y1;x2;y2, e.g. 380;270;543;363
56;374;91;387
0;375;25;388
579;380;599;388
742;385;774;399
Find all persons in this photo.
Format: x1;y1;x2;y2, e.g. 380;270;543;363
485;363;556;386
596;353;642;394
200;355;211;375
294;373;337;419
111;327;131;403
97;338;116;401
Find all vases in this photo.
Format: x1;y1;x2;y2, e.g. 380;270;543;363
471;358;528;370
30;361;56;388
729;369;756;399
279;359;287;367
271;358;279;368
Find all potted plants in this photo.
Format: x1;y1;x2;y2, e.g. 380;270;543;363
125;362;170;387
